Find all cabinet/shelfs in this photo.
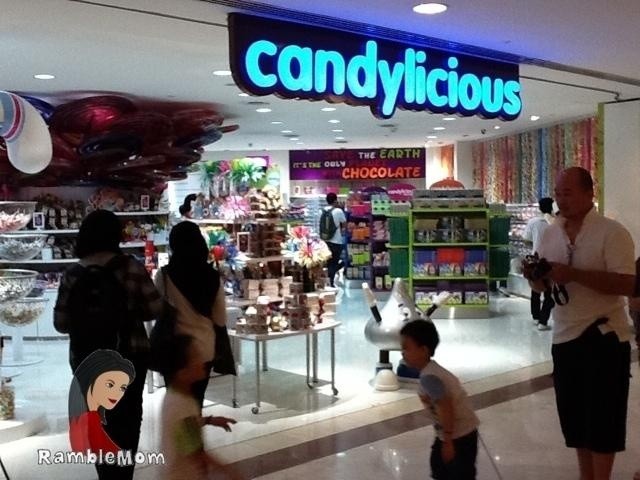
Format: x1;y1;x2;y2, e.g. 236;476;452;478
193;216;294;307
1;198;172;339
340;205;541;317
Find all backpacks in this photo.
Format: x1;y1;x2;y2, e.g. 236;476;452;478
317;206;340;240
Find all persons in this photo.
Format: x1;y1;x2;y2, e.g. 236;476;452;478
324;192;347;287
400;319;480;480
67;348;135;455
54;209;162;480
523;197;552;329
179;194;197;218
149;220;225;417
155;334;237;480
521;166;637;480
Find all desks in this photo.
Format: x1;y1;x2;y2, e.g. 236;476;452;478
229;321;342;414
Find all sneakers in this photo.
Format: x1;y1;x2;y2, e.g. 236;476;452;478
532;318;552;332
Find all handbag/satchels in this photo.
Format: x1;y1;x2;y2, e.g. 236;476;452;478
144;266;177;376
213;320;237;377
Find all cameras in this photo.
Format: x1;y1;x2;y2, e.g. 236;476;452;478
523;251;551;282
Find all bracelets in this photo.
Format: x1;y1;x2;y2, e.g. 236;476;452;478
204;415;212;423
443;431;453;436
